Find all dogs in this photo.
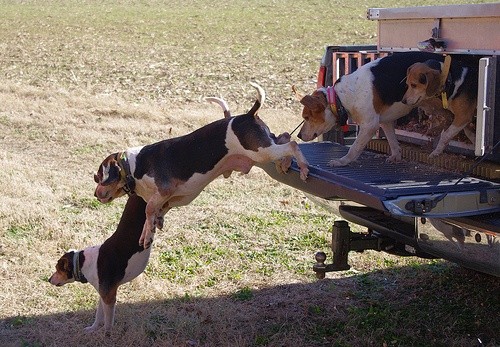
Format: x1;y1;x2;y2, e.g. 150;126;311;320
48;193;169;339
401;62;479;159
296;50;458;167
93;81;310;250
